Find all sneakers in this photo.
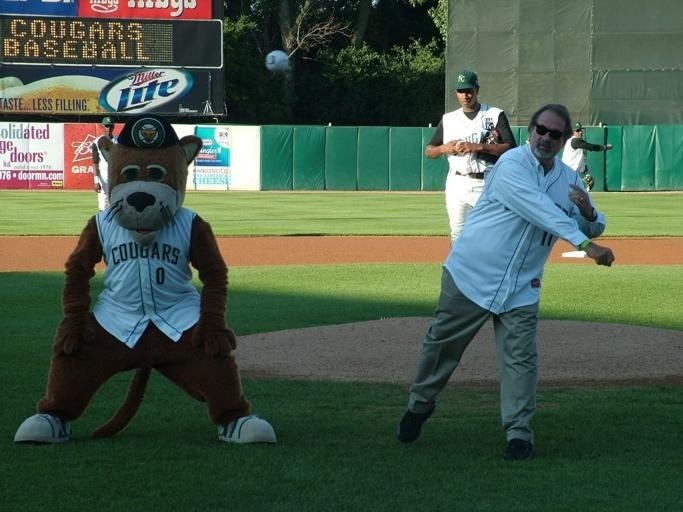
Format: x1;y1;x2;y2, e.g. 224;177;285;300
398;404;435;442
504;439;532;461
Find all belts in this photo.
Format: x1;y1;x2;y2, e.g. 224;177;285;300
450;172;485;178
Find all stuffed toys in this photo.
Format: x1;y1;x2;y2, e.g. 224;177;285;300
13;110;278;450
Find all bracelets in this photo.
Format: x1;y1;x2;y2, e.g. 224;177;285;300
93;175;99;184
602;144;606;150
578;237;594;253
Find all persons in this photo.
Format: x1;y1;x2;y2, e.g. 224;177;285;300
396;103;613;459
422;68;518;246
88;116;121;209
561;121;612;192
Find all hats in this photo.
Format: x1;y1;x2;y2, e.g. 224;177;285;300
455;69;479;90
102;117;114;126
572;122;585;131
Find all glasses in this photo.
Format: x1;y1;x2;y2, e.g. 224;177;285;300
576;129;581;132
105;124;114;128
535;122;565;140
